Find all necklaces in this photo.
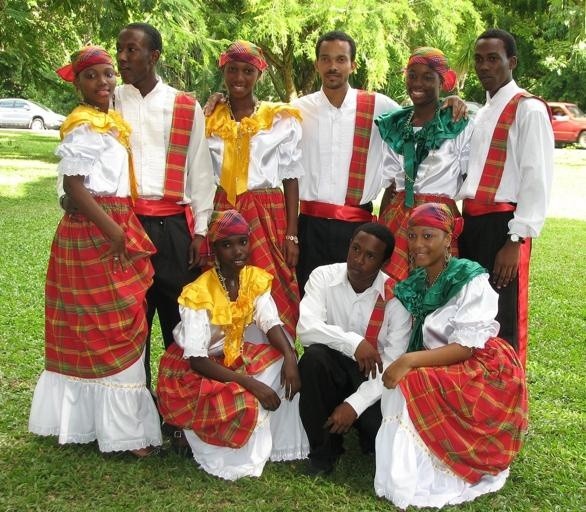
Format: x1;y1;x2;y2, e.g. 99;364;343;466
425;272;442;291
400;111;437;184
218;267;229;293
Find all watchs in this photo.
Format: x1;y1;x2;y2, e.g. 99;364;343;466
284;235;299;245
507;234;526;245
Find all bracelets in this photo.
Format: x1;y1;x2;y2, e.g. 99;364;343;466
61;194;65;208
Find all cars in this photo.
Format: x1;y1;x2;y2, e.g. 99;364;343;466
545;101;585;149
0;98;66;130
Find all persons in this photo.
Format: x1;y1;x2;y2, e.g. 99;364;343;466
155;209;311;481
204;41;303;344
374;203;529;509
203;32;468;301
56;23;218;387
295;222;413;481
375;46;476;281
458;29;555;371
27;46;166;459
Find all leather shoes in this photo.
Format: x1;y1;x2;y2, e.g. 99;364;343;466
164;423;193;459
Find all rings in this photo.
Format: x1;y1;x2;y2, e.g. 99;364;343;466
113;255;119;260
